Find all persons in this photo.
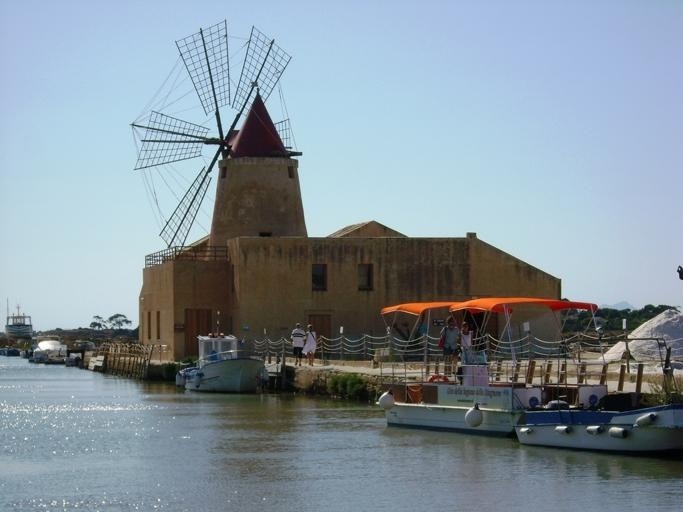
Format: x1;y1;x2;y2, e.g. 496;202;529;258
290;322;305;366
456;321;473;348
301;324;318;366
440;315;459;377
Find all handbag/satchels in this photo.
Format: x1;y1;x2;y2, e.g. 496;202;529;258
438;335;446;348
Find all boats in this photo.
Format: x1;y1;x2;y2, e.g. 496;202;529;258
174;309;266;395
511;337;682;453
372;299;599;438
0;297;71;365
